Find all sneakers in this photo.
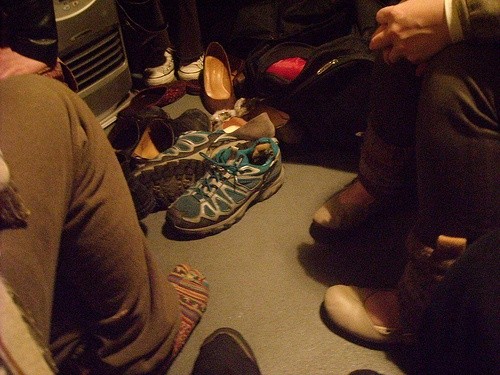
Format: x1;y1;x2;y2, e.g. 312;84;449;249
166;136;287;235
131;128;238;220
177;52;207;81
144;47;176;87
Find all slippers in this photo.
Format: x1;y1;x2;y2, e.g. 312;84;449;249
116;80;186;118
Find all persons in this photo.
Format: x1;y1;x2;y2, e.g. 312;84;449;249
1;0;59;78
311;0;500;341
1;75;208;374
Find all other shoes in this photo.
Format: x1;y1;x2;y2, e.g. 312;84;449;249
121;109;210;175
107;106;170;162
191;328;260;375
213;112;275;140
184;80;201;96
201;42;235;114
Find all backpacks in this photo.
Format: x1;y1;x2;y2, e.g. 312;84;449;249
253;35;376;151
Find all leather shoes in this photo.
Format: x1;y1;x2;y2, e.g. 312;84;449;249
312;179;380;232
322;285;418;347
